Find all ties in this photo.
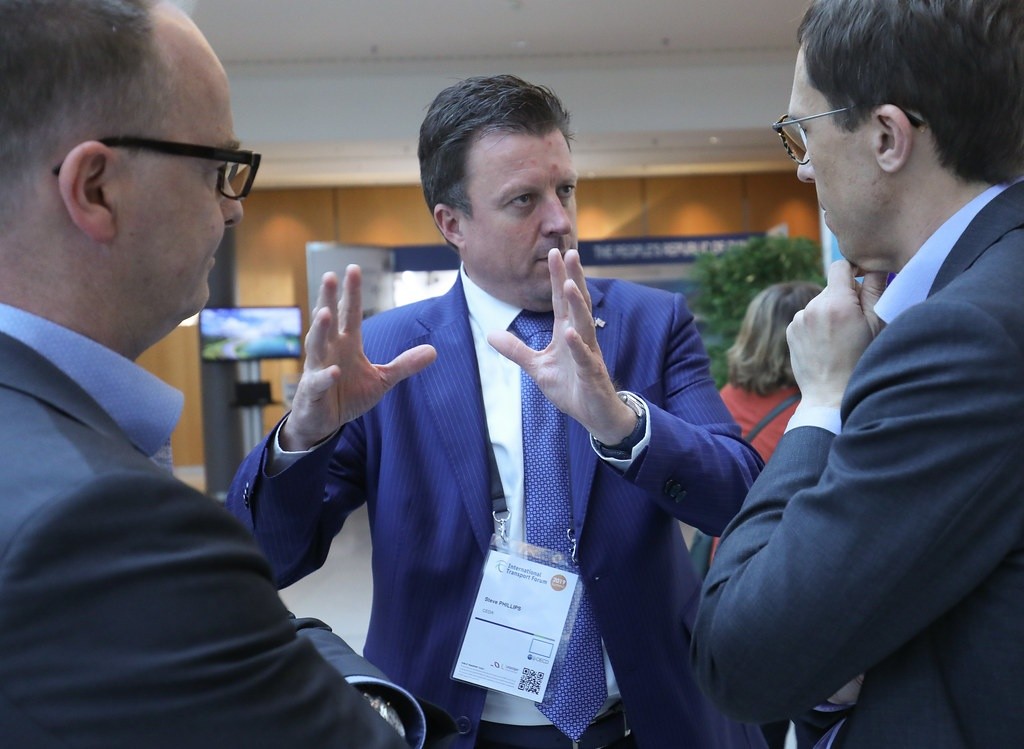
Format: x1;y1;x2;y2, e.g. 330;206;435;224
772;100;927;165
510;309;608;742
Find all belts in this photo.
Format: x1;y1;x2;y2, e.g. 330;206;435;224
478;710;630;749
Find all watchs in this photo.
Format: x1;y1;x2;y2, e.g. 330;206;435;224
365;693;407;740
592;395;646;459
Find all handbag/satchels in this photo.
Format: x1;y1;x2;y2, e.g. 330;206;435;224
688;526;714;581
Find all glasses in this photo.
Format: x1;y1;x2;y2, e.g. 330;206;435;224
54;137;261;201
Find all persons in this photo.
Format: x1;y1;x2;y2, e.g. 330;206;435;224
709;283;830;749
690;0;1023;749
0;0;458;749
226;74;764;749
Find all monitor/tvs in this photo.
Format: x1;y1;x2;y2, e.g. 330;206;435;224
199;306;302;363
